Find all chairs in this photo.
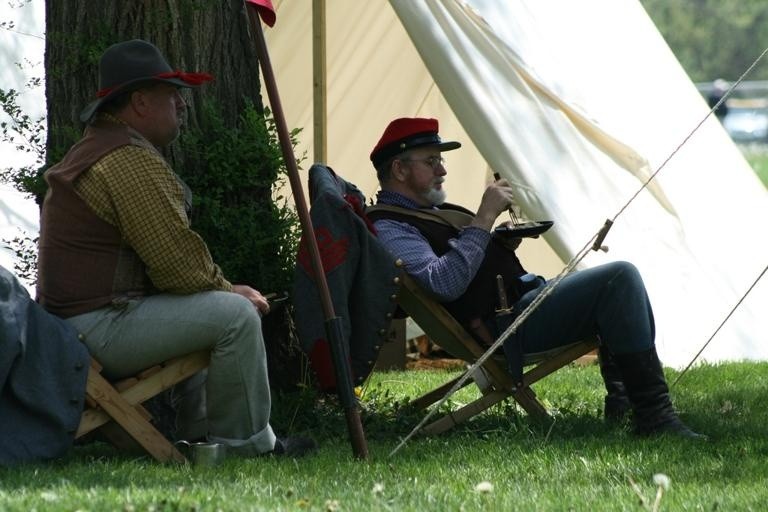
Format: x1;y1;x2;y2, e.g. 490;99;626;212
0;265;211;464
307;163;599;439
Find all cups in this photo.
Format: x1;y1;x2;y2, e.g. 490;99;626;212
172;439;226;466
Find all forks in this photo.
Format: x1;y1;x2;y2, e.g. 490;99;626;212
492;172;519;226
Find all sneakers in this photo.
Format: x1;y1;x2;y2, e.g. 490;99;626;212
258;437;317;459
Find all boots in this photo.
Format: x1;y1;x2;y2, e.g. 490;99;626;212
612;346;709;443
597;344;632;425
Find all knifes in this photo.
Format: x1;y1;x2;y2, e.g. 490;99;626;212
494;274;525;389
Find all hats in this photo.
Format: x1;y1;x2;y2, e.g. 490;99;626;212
79;38;196;122
370;118;461;169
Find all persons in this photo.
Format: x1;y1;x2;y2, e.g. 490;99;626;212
35;38;313;459
369;116;712;440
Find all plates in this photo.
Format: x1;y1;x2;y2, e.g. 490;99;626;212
493;220;555;239
